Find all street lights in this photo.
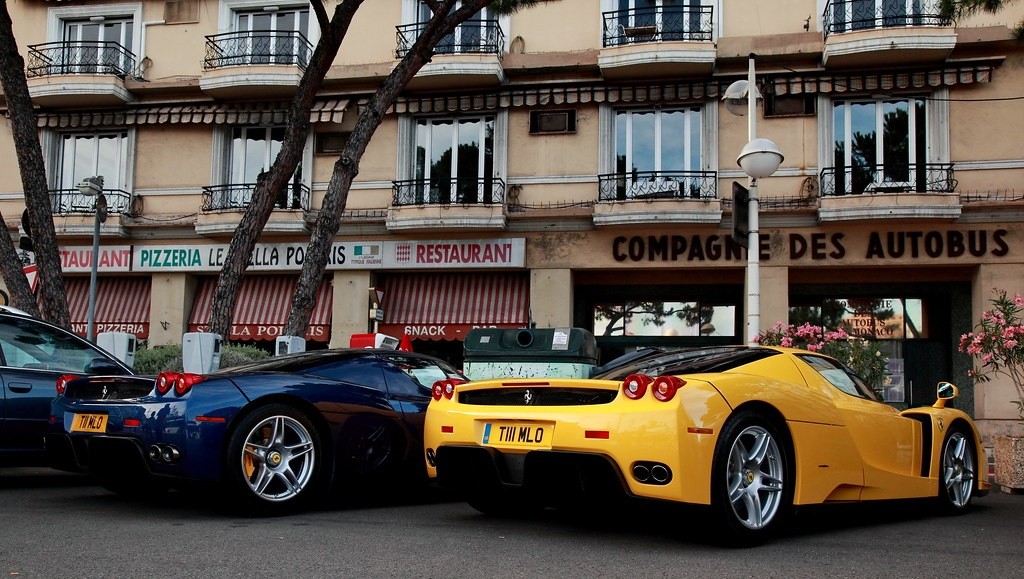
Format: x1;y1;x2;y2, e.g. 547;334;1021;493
720;53;784;348
76;174;106;351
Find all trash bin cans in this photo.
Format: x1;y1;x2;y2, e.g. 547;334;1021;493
463;326;599;380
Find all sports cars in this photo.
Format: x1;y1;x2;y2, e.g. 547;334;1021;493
44;348;497;517
422;342;986;548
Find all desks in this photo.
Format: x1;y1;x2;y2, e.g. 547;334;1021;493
624;25;659;42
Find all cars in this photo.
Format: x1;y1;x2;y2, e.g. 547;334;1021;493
0;311;156;470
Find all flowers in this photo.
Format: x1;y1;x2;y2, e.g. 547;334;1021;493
752;322;894;391
958;285;1024;427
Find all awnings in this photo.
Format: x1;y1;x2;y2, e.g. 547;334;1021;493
379;271;527;339
188;274;336;341
28;273;152;336
